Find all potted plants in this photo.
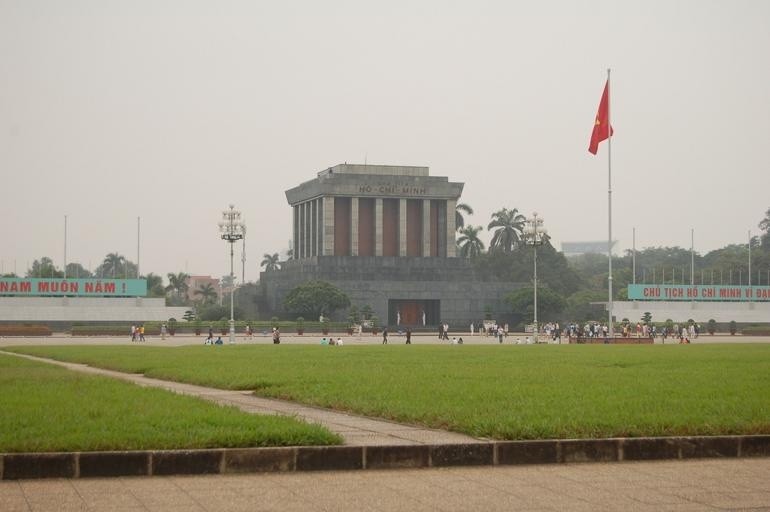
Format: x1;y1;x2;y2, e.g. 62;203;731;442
271;316;279;333
345;316;355;335
708;319;716;336
219;316;230;335
296;316;305;335
194;318;202;335
370;316;379;335
729;320;737;335
320;316;329;334
244;318;254;334
167;318;178;335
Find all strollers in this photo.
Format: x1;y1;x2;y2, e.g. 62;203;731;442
680;335;690;344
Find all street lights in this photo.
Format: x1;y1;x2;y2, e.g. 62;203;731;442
217;203;246;346
522;210;549;343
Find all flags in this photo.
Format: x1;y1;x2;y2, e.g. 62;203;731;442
587;80;613;155
